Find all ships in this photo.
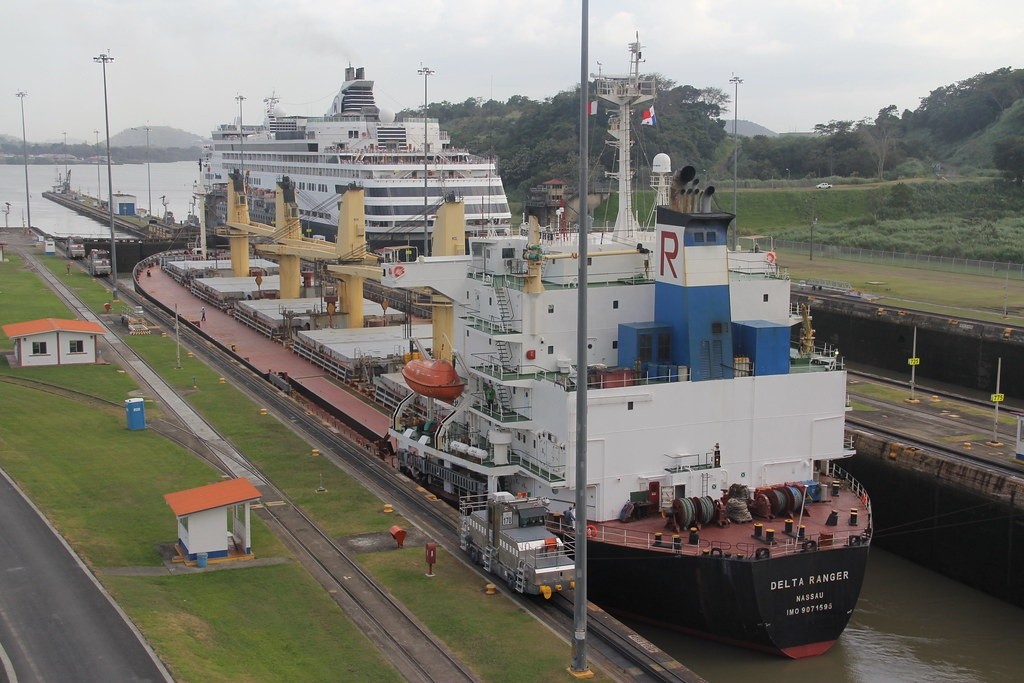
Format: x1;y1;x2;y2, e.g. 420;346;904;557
130;32;876;661
185;65;513;261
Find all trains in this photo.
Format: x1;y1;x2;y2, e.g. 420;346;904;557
65;236;85;260
458;491;576;597
87;248;112;277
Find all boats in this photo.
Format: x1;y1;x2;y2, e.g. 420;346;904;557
400;357;466;403
246;187;276;200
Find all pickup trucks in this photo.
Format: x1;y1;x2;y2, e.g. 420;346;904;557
816;182;832;190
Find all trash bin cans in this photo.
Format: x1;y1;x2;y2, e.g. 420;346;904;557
197;553;208;568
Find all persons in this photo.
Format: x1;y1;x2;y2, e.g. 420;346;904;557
563;504;576;531
199;307;206;321
486;384;496;413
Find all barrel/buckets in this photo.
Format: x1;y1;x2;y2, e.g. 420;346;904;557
197;553;207;567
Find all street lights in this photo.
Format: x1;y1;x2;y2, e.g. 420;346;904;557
733;80;745;250
61;132;68;195
92;129;102;209
143;128;153;223
234;95;246;190
786;169;790;180
419;68;437;257
93;54;118;304
14;89;31;235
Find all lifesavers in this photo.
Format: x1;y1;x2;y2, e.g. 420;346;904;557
587;524;598;537
766;251;776;264
392;264;407;280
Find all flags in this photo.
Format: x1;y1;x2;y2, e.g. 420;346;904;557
640;105;657;126
588;100;598;115
